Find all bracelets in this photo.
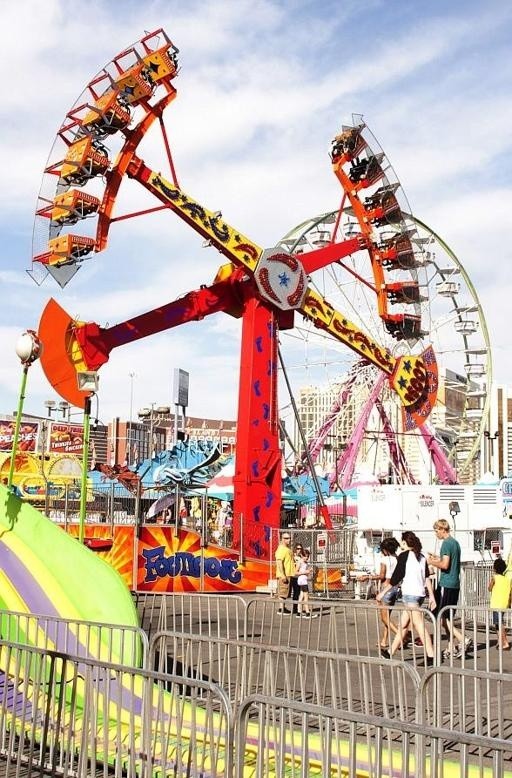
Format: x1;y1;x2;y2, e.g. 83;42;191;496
369;575;372;580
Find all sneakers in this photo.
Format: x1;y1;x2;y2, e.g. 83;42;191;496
417;656;433;667
442;649;458;659
458;639;471;659
381;649;390;659
296;611;318;620
278;607;292;615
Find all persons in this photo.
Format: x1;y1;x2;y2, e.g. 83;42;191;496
224;511;233;525
379;531;433;667
291;543;303;614
488;559;512;650
274;533;294;616
295;550;318;619
333;133;415;341
355;537;401;647
428;518;472;659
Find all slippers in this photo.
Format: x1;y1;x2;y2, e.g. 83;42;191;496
398;641;410;650
376;642;387;647
496;644;510;650
